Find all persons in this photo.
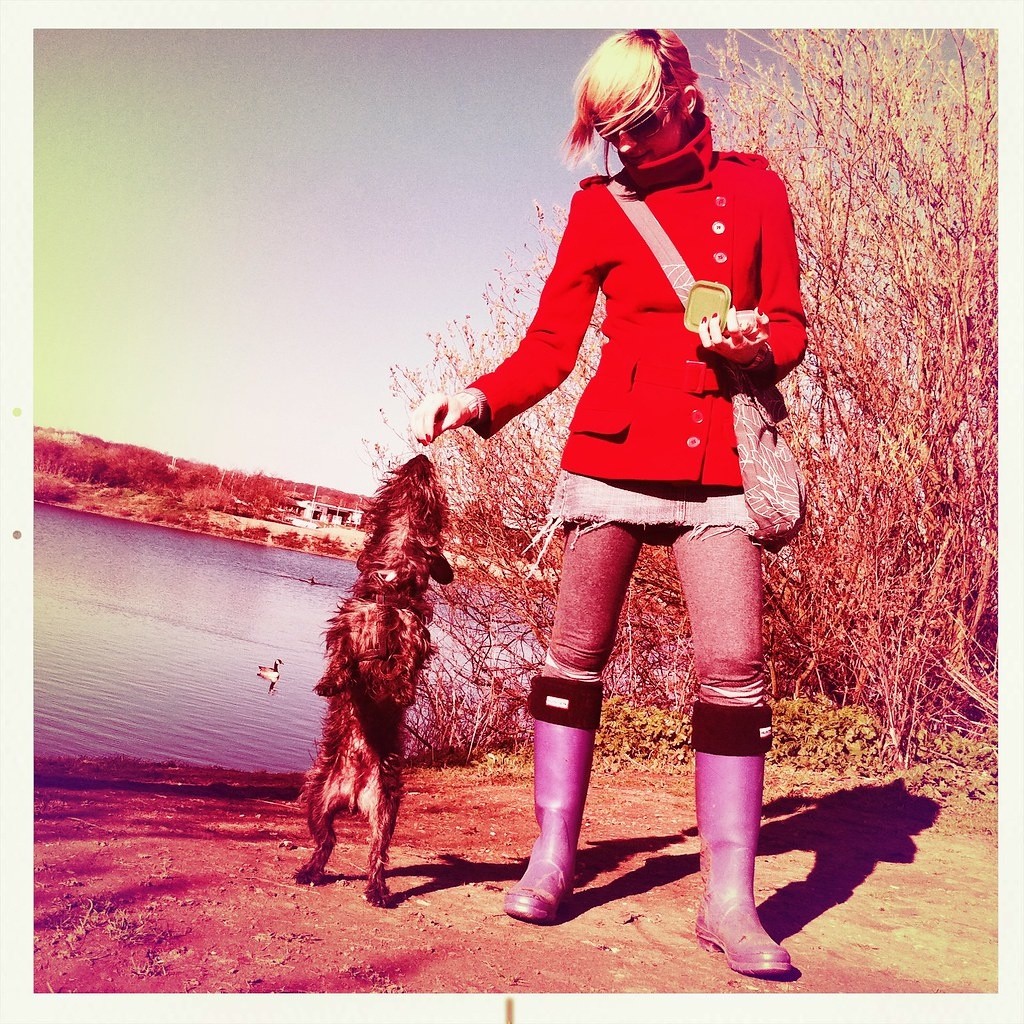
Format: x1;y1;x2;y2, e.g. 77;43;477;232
411;29;806;975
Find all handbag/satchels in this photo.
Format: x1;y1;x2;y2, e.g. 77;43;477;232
728;372;809;546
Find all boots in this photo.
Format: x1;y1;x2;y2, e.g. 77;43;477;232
501;673;604;926
689;694;796;976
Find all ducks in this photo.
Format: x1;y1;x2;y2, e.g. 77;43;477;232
258;659;285;679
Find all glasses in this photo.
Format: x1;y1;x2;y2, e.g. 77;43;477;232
592;90;683;142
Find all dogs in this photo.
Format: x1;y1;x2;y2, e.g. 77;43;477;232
291;454;453;910
